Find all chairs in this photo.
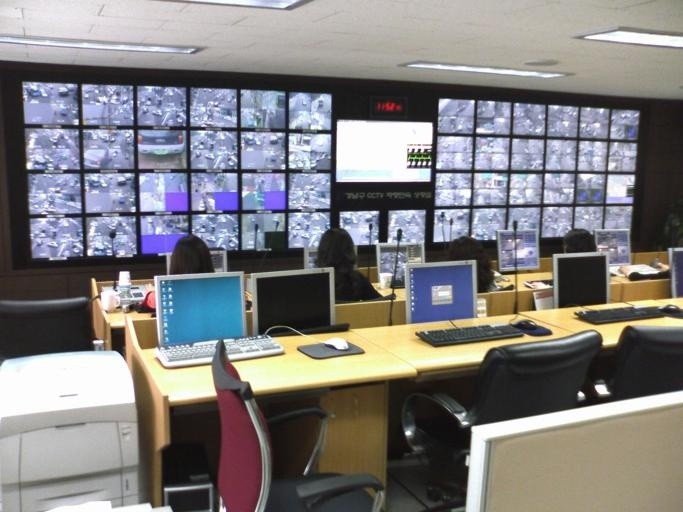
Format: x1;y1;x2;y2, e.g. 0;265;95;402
0;293;94;368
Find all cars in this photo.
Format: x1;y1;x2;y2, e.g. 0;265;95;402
338;212;428;284
433;96;641;270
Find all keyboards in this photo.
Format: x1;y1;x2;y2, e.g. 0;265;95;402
155;334;284;368
415;324;524;347
574;306;665;325
524;280;553;291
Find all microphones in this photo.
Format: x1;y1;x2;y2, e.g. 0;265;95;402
449;218;453;243
253;224;259;252
366;223;372;283
388;229;402;325
274;222;280;233
513;219;518;315
109;230;117;292
440;212;446;244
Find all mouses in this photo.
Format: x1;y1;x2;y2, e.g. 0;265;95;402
324;338;349;351
664;304;680;312
324;336;349;351
517;319;537;330
512;319;537;329
666;304;680;313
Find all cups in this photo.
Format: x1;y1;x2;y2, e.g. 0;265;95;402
118;270;132;286
101;291;121;312
378;272;393;289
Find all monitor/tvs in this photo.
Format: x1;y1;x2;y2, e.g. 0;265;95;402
552;252;610;308
385;209;427;244
434;172;473;208
470;97;641;242
668;247;683;298
21;81;334;217
239;212;285;251
435;135;475;172
374;243;426;286
250;267;336;335
335;118;433;184
139;214;191;256
27;214;83;261
264;230;288;250
84;214;139;257
339;210;379;248
190;212;239;252
222;247;309;272
594;228;632;267
432;207;471;242
436;97;476;135
496;229;539;275
164;249;228;275
405;260;477;324
287;212;332;252
153;271;248;346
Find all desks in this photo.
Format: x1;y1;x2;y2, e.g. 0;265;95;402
81;249;681;512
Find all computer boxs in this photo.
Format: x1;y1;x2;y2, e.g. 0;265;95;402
162;419;214;512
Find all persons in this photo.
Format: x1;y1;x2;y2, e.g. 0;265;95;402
447;234;498;293
317;228;385;303
144;235;215;315
563;228;598;252
